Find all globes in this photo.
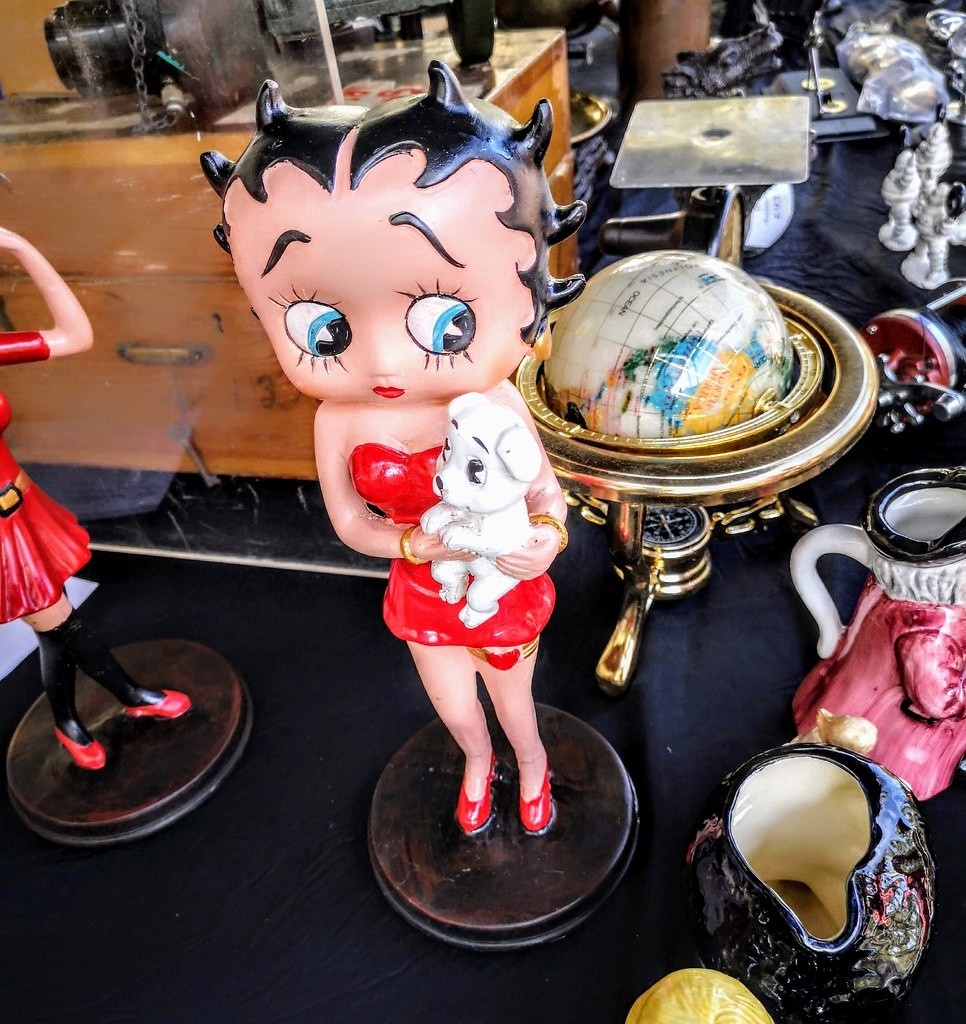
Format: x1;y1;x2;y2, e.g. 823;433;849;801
514;246;879;693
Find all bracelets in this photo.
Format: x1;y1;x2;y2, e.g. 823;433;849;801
400;526;431;565
531;515;569;554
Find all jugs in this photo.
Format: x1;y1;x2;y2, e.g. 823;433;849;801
679;740;937;1024
791;467;966;801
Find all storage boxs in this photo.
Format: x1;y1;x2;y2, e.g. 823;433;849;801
0;27;580;484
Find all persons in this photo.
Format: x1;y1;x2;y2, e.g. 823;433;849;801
200;57;589;835
0;227;192;773
